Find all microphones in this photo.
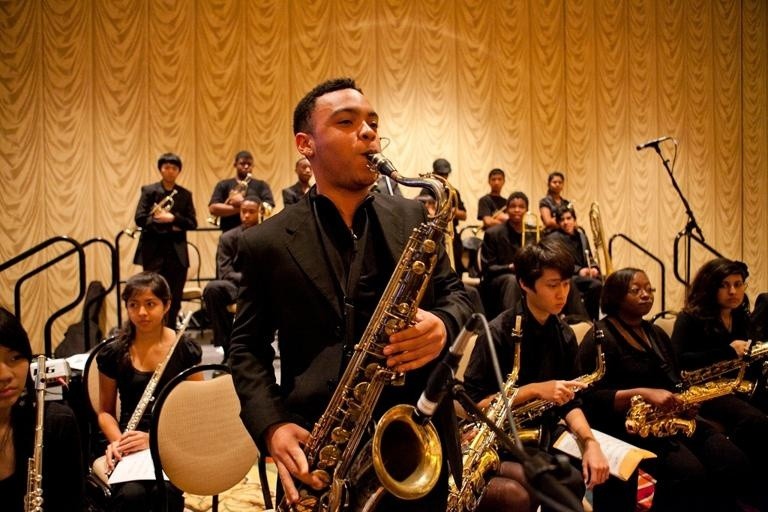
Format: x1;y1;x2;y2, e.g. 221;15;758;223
409;311;485;427
636;135;670;152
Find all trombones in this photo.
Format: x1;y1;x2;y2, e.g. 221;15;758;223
585;201;614;283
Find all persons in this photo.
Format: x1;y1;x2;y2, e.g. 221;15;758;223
667;256;768;510
449;395;540;511
373;159;607;380
87;272;205;511
0;304;91;510
229;77;475;510
128;147;313;379
578;267;739;511
474;236;637;511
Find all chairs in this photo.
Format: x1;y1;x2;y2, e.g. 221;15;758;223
476;242;484;273
452;332;485;419
563;315;595;346
650;310;679;339
179;240;204;337
82;335;129;512
148;362;275;512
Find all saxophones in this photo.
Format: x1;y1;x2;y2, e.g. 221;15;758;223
445;315;524;512
624;337;758;439
461;320;606;454
276;153;460;512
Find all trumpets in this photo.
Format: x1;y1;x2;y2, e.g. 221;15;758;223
124;189;178;239
207;172;253;225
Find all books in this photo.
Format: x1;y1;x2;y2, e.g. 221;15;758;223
553;425;661;482
107;440;170;486
